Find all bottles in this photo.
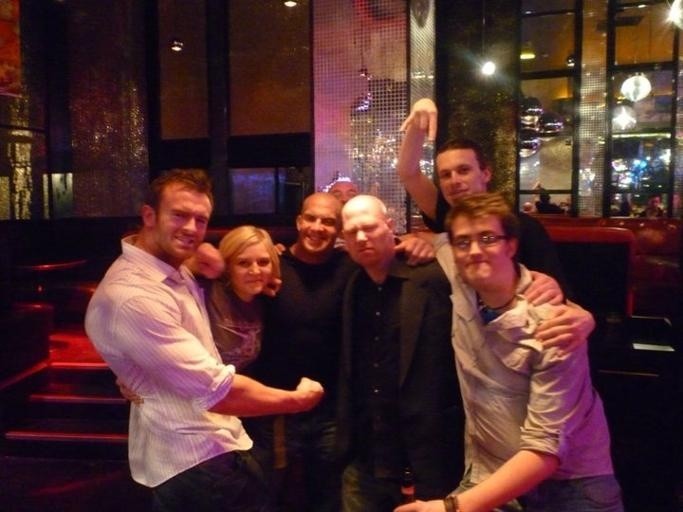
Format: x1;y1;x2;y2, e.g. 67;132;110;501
400;471;415;503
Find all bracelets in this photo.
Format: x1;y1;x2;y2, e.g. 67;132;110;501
443;493;459;512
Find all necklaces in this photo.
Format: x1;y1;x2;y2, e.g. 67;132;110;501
478;293;517;310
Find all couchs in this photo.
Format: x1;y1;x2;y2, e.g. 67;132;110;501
526;217;683;293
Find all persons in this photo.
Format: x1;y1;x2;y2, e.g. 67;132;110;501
391;189;625;512
113;221;281;510
521;201;534;213
670;192;682;217
275;194;597;512
396;96;601;359
531;181;566;213
84;168;327;511
635;194;664;217
613;201;634;217
323;173;358;206
266;192;436;512
563;193;572;213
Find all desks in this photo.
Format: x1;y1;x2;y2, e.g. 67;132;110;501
9;261;88;300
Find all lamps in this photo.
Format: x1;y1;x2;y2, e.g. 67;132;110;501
520;46;536;60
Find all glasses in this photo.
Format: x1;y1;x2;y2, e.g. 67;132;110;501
454;234;509;249
